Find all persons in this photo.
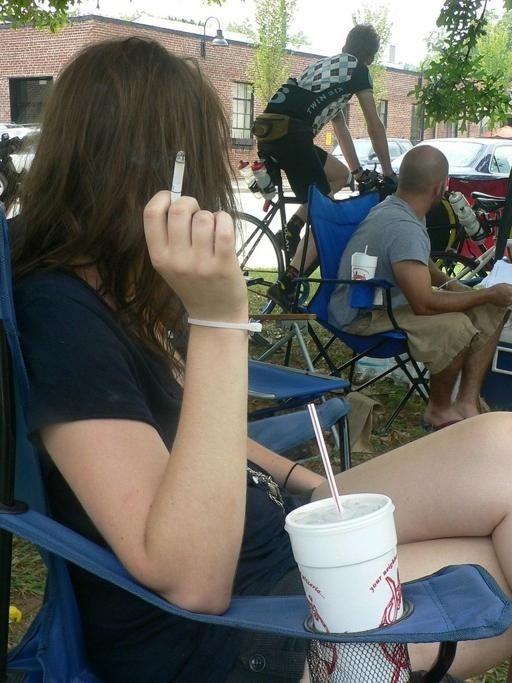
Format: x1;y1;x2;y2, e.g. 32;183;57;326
330;145;512;429
0;35;512;681
247;24;396;315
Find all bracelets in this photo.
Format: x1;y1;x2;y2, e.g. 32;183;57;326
183;317;264;334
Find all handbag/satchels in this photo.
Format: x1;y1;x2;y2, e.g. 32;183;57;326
248;113;310;139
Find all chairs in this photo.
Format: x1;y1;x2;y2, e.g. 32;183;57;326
171;311;352;426
282;184;477;436
0;201;512;683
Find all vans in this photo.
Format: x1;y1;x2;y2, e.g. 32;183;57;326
333;137;414;177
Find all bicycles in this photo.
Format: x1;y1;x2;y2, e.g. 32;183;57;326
446;189;512;291
232;162;458;332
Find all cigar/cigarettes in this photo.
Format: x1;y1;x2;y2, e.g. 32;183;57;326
164;148;189;197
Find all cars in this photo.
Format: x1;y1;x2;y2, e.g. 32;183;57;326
384;138;512;187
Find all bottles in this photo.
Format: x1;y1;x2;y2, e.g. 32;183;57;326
238;159;261;193
458;205;486;245
449;190;469;214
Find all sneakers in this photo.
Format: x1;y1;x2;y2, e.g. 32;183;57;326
274;223;301;256
266;279;307;314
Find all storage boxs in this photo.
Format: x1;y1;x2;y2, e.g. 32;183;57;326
484;323;512;407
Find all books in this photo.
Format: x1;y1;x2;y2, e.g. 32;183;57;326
484;259;511;287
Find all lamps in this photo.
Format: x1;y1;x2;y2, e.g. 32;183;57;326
199;13;230;60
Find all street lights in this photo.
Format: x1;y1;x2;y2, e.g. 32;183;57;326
201;17;229;58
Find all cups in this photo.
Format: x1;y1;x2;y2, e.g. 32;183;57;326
236;160;255;186
350;253;378;305
284;492;410;682
445;191;486;246
